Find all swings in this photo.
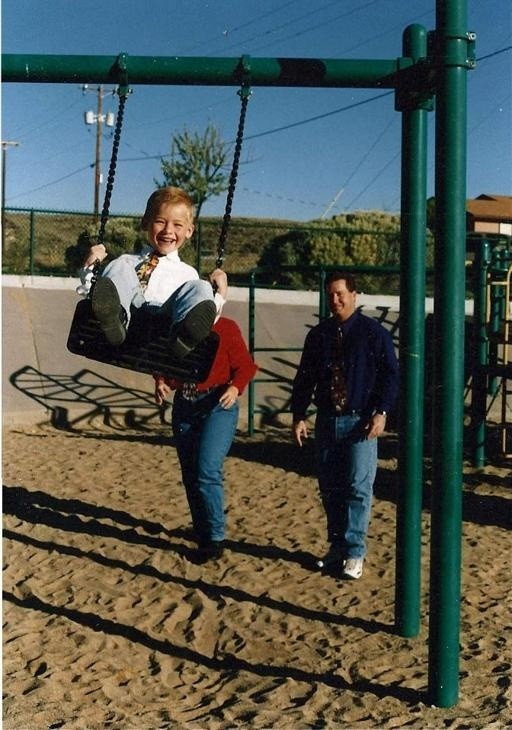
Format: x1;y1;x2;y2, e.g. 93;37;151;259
66;53;253;383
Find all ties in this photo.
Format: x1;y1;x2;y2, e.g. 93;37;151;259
328;326;349;415
135;252;160;296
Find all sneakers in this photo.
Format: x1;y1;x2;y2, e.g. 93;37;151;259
314;546;364;579
170;298;217;362
189;541;226;566
90;274;129;349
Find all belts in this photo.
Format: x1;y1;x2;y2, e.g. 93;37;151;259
321;410;366;417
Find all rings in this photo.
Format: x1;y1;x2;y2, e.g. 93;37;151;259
230;399;233;402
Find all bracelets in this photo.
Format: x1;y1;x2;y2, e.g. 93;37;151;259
373;408;388;416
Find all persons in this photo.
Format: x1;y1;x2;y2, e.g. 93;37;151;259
75;185;231;357
153;274;258;561
289;272;400;580
